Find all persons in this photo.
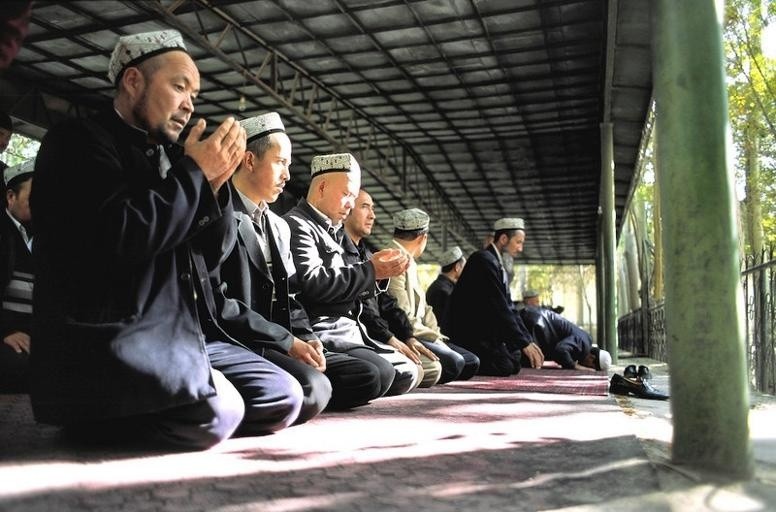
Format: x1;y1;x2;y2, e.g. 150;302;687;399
209;110;381;430
428;245;467;317
282;152;419;399
1;162;39;395
0;115;15;171
444;212;564;377
377;207;480;385
342;191;442;388
504;302;611;375
33;30;303;452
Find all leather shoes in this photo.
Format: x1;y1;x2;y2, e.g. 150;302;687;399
609;373;670;400
639;365;653;379
625;365;637;378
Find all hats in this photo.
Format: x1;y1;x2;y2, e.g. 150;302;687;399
107;29;188;83
3;159;36;183
239;111;285;143
522;290;538;297
392;208;429;231
438;245;463;267
310;153;362;177
600;350;612;369
494;218;524;230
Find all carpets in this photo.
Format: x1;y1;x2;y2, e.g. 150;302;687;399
443;361;610;396
